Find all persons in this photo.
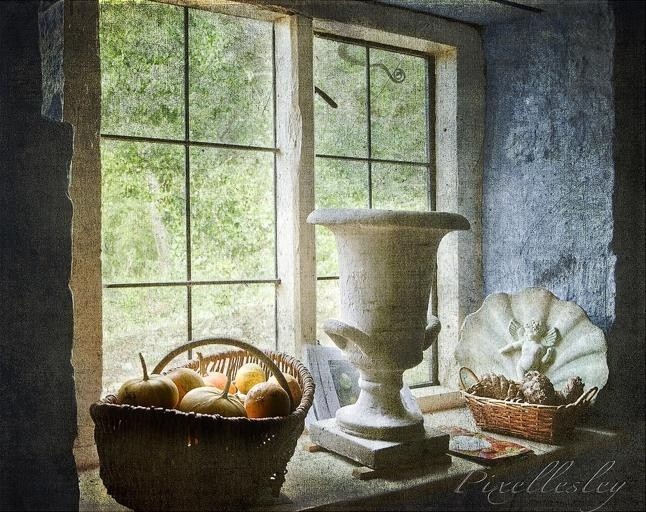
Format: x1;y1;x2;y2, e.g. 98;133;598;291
502;317;556;383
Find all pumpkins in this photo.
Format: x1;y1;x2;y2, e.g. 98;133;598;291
118;352;302;418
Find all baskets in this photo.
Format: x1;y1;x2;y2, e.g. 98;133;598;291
459;365;598;443
89;336;316;512
